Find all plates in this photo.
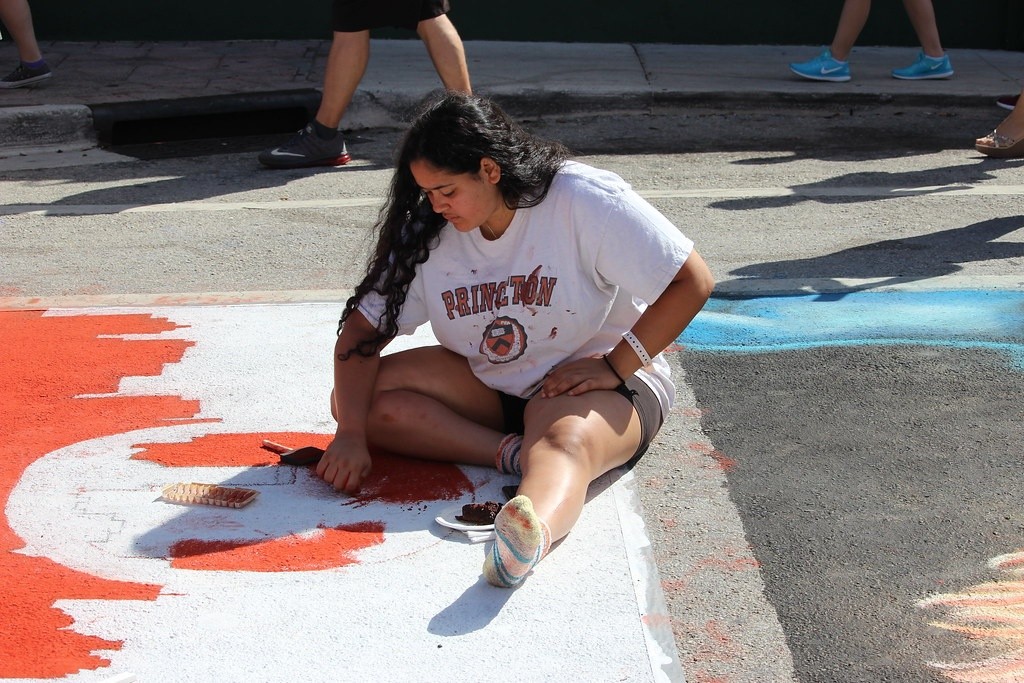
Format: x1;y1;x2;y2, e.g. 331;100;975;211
435;504;495;531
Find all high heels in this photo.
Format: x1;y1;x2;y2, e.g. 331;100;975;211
976;125;1024;156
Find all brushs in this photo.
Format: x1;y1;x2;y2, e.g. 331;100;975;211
260;438;326;467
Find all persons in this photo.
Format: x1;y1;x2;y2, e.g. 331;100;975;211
317;90;717;588
1;1;1022;170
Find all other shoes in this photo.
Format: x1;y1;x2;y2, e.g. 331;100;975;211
0;62;53;88
995;94;1021;110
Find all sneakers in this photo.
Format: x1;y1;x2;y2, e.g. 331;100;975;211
258;122;351;168
789;48;851;81
890;49;954;80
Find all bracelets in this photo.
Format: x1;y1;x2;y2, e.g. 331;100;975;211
621;331;653;368
602;353;626;385
